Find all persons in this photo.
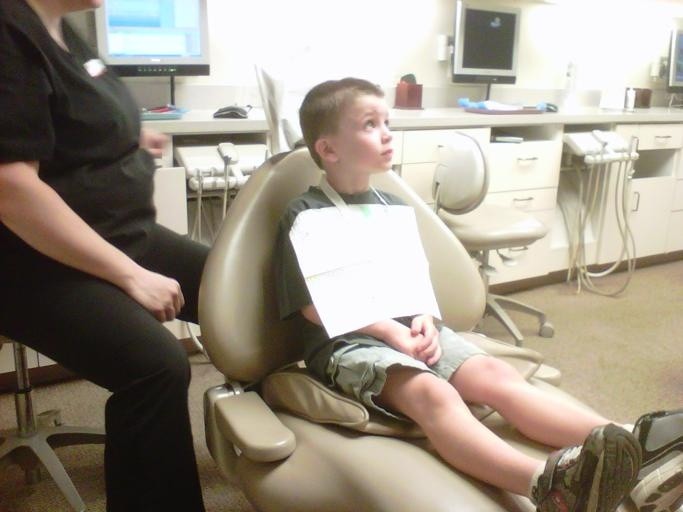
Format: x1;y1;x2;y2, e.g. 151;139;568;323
273;77;683;512
0;0;211;512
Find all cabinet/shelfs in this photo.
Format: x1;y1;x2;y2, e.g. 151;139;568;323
386;123;683;285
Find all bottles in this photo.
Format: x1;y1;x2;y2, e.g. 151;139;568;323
626;88;636;109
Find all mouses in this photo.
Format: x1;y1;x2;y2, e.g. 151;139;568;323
213;105;247;120
546;103;558;113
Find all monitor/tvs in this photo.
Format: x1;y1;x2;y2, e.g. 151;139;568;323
93;1;211;78
665;27;683;93
451;0;522;83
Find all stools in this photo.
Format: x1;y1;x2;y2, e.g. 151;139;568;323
0;327;107;512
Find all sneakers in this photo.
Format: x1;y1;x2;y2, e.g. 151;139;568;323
626;410;681;511
536;423;643;512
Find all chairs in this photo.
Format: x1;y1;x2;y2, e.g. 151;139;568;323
433;132;555;346
196;147;683;512
